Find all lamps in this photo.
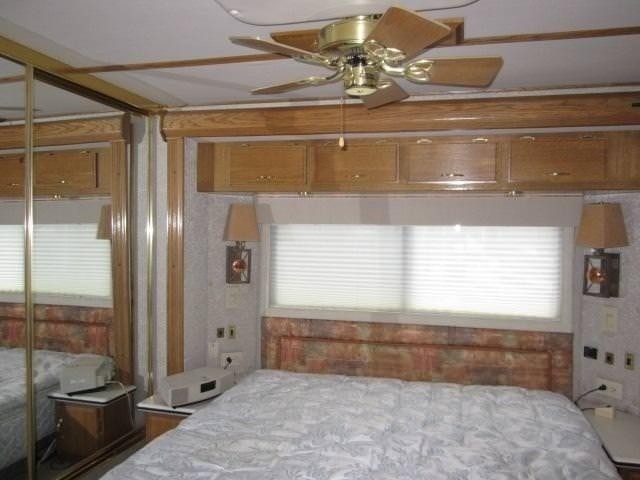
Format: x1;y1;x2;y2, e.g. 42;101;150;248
94;202;113;241
574;202;630;301
220;202;260;286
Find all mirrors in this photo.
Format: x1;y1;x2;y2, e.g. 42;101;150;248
1;50;140;476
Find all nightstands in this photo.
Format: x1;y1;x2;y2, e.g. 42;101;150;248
41;386;136;456
136;392;203;443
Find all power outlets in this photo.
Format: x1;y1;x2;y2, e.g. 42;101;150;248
221;352;241;368
596;375;609;396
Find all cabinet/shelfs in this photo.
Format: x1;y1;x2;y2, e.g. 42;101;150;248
0;149;113;196
197;130;640;192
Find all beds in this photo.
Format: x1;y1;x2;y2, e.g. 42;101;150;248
99;315;625;480
2;340;113;468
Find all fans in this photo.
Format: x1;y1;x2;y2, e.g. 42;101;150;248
226;9;505;115
